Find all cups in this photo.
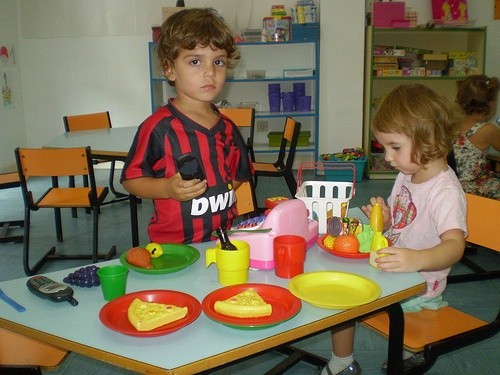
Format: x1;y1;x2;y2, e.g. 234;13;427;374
98;265;129;302
270;82;311;112
152;26;163;40
273;235;307;279
205;241;251;285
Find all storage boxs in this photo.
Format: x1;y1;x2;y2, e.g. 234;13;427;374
295;181;353;234
370;2;411;28
151;24;161;42
161;7;192;23
267;131;311;147
431;0;469;24
371;152;394;170
263;16;291;42
292;22;320;40
320;154;367;182
372;44;480;77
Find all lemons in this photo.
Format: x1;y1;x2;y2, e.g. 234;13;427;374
145;242;163;258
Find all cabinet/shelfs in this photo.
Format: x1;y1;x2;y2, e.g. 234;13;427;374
363;26;488;179
148;38;320;175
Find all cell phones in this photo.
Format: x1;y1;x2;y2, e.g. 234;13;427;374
27;276;77;305
176;151;205;183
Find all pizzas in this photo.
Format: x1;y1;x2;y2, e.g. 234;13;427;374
128;298;188;331
214;288;272;318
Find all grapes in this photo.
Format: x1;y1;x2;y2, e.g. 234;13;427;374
63;266;100;287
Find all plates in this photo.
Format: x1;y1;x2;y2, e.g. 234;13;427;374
99;290;202;338
202;283;303;330
288;270;381;309
120;243;200;274
318;233;392;257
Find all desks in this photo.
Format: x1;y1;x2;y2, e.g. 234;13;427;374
43;126;138;243
0;241;427;375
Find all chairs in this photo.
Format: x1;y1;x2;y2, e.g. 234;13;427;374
252;116;302;213
63;110;142;213
0;327;71;375
0;171;30;244
358;194;500;375
235;181;258;220
219;108;259;189
15;145;117;276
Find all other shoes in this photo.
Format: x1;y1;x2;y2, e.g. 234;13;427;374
318;359;362;375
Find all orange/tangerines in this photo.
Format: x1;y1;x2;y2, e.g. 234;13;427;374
334;234;359;254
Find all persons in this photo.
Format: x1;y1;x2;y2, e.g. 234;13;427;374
319;83;469;374
119;8;254;244
449;75;500;202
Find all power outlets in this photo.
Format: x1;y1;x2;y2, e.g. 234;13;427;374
257;121;268;132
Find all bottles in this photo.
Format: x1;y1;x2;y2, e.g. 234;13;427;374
217;228;237;250
